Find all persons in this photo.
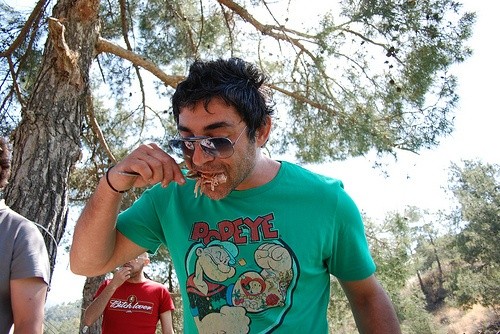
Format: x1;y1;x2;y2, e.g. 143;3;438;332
0;136;50;334
68;57;402;334
84;250;177;334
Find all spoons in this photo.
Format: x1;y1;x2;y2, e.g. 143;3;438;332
117;170;203;180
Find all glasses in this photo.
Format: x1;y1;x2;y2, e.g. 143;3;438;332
134;256;145;261
166;124;248;158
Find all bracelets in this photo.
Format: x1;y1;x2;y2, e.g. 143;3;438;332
106;165;132;193
